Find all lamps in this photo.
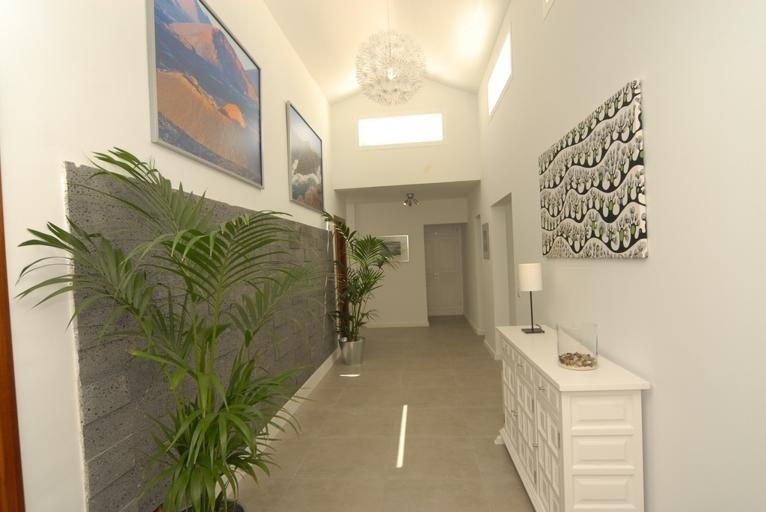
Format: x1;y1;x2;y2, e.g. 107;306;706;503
355;0;426;107
517;262;547;334
399;192;424;209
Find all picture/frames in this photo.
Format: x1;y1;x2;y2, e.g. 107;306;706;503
148;0;263;190
285;100;324;215
377;235;409;263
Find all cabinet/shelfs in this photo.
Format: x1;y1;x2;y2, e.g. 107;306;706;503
491;324;653;512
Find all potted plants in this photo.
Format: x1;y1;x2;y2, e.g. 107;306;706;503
317;211;398;365
13;142;306;512
